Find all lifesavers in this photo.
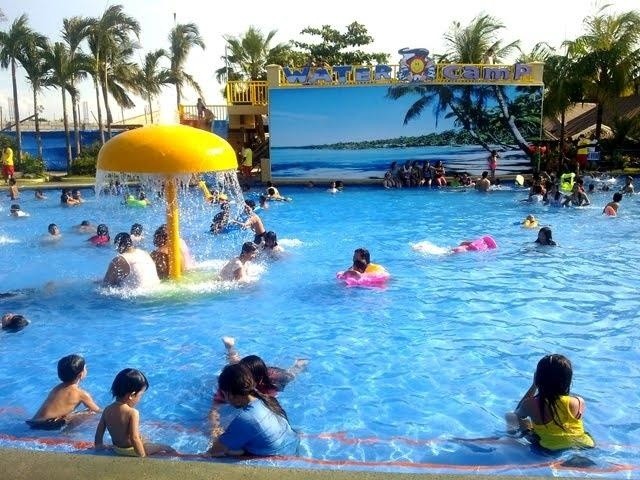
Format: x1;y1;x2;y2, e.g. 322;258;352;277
126;194;146;207
198;179;227;199
337;270;390;289
452;236;497;252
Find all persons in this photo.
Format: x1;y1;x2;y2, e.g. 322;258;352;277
327;182;341;193
93;369;177;458
535;228;556;245
381;148;636;217
353;261;366;273
35;189;46;198
80;220;191;286
561;133;601;172
208;181;281;281
109;180;146;201
26;355;102;432
209;364;299;455
239;142;252;176
10;204;24;216
61;186;83;205
523;214;538;227
3;142;15;185
1;312;29;333
515;353;595;454
306;180;313;190
48;223;58;236
9;177;18;199
353;249;386;274
213;336;310;406
197;98;214;121
